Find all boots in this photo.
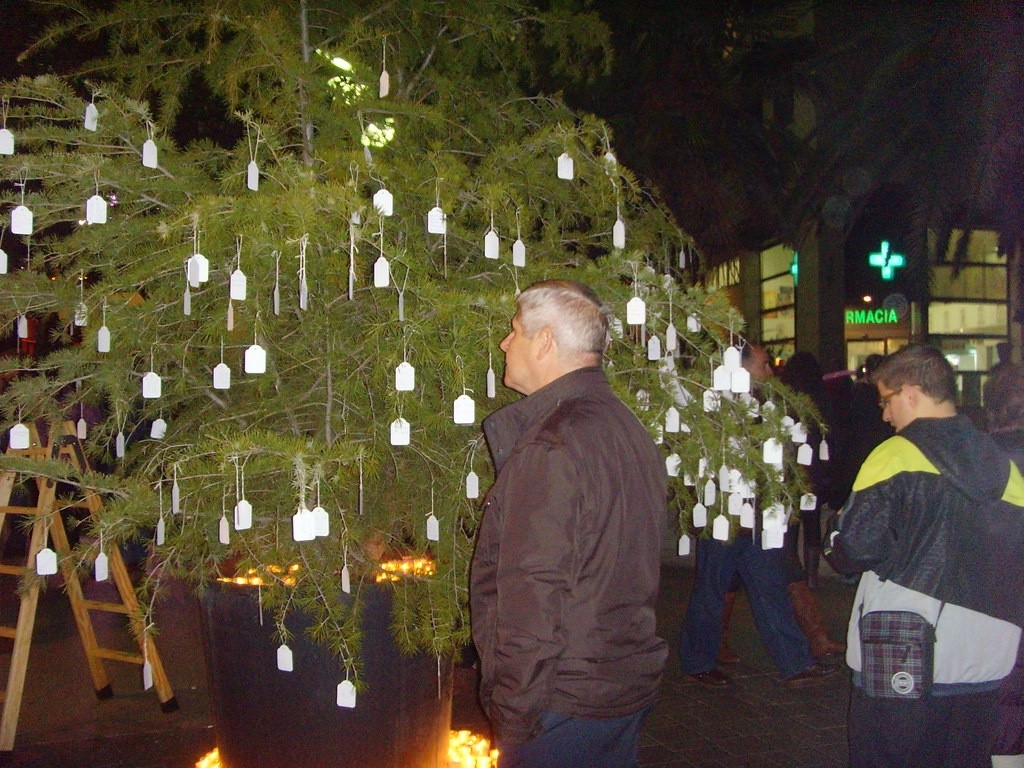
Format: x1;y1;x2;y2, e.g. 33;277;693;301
717;592;740;663
803;545;819;587
790;581;848;657
789;525;802;565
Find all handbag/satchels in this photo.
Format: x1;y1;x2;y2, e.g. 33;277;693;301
860;610;931;700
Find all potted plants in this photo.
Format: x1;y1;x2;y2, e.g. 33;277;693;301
0;0;831;768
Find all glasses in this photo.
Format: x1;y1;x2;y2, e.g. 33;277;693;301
878;390;900;409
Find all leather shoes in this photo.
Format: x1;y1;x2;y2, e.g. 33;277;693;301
684;670;734;689
787;665;839;689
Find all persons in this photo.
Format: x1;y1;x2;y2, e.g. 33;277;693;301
676;342;1024;692
468;279;669;767
821;341;1024;768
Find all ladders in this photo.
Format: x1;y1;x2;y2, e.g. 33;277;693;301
1;413;192;753
